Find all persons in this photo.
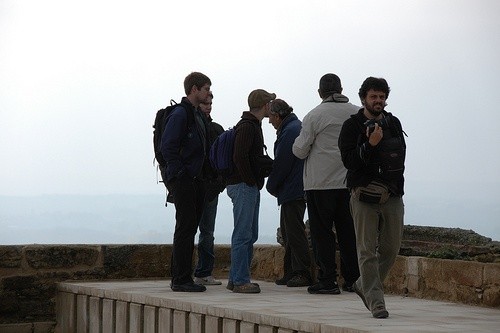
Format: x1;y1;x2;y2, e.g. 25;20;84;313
266;98;311;287
338;77;407;318
194;90;225;285
160;72;212;293
226;89;276;293
292;73;361;295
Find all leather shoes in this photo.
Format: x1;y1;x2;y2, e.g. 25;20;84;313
170;279;205;292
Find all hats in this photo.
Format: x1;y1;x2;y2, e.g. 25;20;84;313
248;89;276;108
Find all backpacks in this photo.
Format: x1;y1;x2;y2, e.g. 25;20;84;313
153;99;195;203
351;111;408;181
207;120;268;177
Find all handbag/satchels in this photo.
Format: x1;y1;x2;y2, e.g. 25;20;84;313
354;182;389;206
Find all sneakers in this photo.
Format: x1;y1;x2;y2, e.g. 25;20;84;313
193;274;221;286
307;284;339;295
353;280;389;319
227;281;261;293
276;272;319;287
342;283;359;292
